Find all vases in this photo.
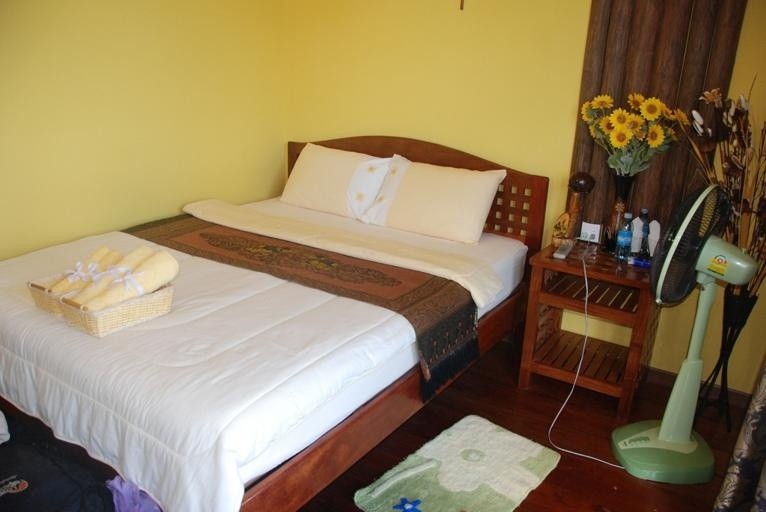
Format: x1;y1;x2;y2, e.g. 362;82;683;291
603;175;636;247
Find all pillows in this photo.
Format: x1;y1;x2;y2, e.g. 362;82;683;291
280;141;393;221
358;153;507;246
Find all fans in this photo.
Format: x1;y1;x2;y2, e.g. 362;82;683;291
612;183;758;484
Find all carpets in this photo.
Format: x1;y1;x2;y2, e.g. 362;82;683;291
355;412;562;512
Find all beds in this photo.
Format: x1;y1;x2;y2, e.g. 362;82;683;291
0;135;550;512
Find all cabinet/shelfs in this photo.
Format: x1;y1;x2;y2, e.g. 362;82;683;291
520;240;663;417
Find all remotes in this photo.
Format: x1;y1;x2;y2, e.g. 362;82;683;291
552;242;573;261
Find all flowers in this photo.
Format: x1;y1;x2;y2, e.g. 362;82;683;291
685;70;766;429
577;94;685;229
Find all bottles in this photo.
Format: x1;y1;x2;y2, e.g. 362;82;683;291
615;212;634;264
639;208;650;261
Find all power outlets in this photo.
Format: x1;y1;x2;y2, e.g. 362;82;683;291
580;222;601;242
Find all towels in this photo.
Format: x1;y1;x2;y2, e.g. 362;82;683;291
48;243;109;295
68;249;121;291
82;250;178;313
75;245;152;301
